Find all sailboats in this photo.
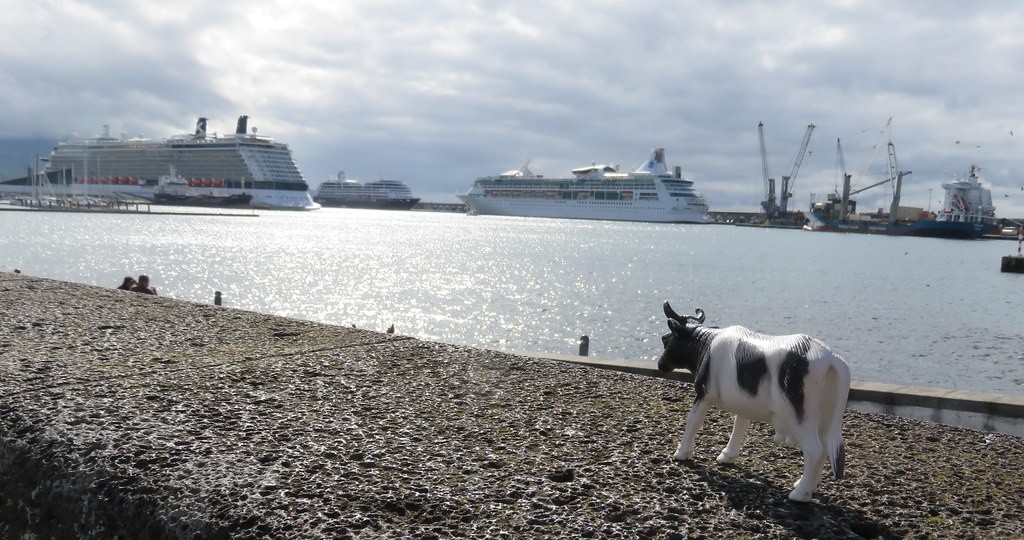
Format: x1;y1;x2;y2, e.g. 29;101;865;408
713;119;1023;239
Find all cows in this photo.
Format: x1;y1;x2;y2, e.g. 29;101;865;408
657;300;851;503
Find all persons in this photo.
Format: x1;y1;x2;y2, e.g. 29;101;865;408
117;277;136;291
129;275;157;295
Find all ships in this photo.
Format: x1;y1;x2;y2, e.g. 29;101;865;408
459;142;713;225
314;168;422;210
2;115;314;209
